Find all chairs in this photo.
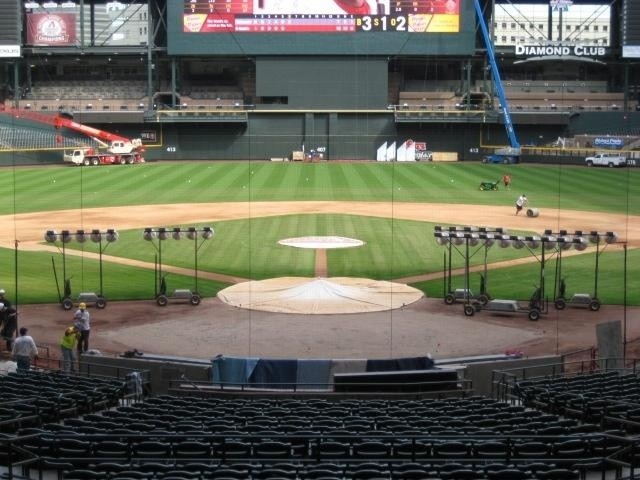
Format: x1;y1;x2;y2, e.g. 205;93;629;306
1;368;640;480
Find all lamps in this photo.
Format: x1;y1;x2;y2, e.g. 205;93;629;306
144;227;215;242
433;225;620;251
44;228;118;244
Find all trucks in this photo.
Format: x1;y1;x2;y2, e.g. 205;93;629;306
482;149;520;163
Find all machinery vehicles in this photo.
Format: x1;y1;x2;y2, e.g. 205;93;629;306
0;103;147;165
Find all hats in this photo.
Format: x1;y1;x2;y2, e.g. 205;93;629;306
79;302;86;310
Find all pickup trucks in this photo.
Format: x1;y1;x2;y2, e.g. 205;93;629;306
585;152;628;168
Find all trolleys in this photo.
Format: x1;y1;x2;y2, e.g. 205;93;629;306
45;228;119;309
142;227;213;306
434;226;618;321
480;175;503;191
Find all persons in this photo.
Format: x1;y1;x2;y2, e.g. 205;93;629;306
72;302;90;352
10;327;38;374
516;193;528;214
60;322;83;372
502;173;510;191
0;289;17;352
